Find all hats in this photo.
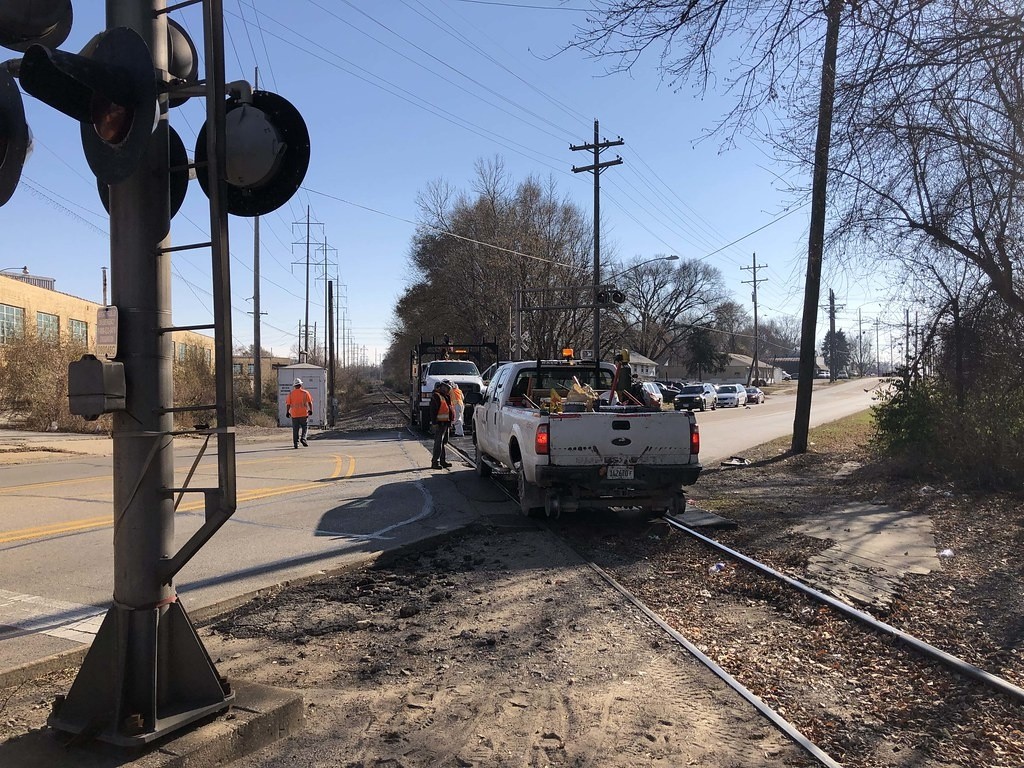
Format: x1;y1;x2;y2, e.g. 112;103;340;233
453;384;458;388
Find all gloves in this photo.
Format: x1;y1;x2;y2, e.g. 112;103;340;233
308;410;313;415
433;424;439;429
286;412;291;418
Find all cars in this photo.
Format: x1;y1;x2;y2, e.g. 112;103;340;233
656;383;685;401
745;386;766;404
782;371;793;381
819;370;830;378
849;369;858;376
717;384;748;407
838;370;848;378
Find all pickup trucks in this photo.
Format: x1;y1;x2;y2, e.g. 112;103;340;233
472;348;701;519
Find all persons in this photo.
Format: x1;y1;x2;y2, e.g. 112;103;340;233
286;378;313;449
429;379;465;470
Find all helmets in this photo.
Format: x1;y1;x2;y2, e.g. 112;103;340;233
442;379;453;389
293;378;303;386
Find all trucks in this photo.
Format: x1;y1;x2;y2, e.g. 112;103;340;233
410;360;512;433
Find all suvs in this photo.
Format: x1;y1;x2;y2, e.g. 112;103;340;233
673;384;718;411
637;382;663;406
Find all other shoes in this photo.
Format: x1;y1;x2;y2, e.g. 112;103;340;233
300;439;308;447
432;462;442;469
440;460;452;467
294;442;299;448
456;434;464;437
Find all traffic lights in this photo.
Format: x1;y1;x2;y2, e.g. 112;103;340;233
598;290;625;304
20;28;159;185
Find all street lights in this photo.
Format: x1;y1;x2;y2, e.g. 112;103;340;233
594;255;680;389
